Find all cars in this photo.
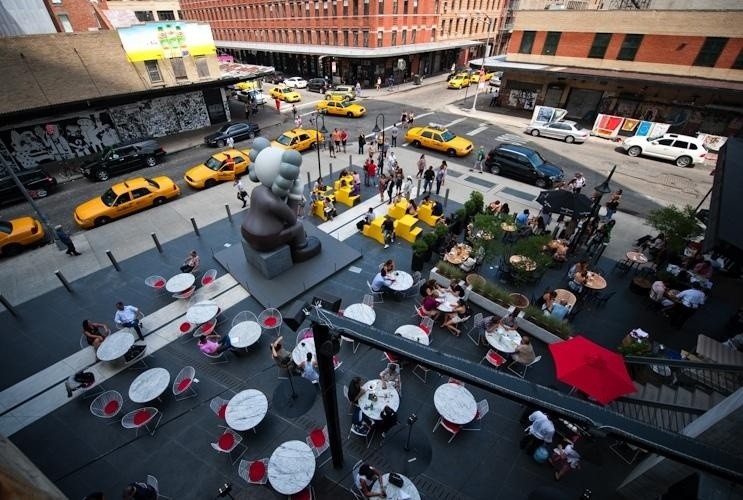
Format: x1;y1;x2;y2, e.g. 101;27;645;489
403;123;473;158
316;95;366;118
470;70;493;83
446;73;470;89
202;122;260;149
489;73;503;87
236;89;267;105
267;83;302;103
282;77;307;89
182;148;251;191
0;215;44;256
270;128;323;150
73;175;180;227
525;120;590;144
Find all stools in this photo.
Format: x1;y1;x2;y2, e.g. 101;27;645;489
310;175;443;247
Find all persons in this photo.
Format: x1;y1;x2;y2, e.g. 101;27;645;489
469;145;485;173
325;72;408;94
650;232;724;328
270;328;340;382
226;135;250;208
226;88;280;121
82;318;110;363
123;480;157;500
53;223;83;256
489;172;623;293
113;302;146;341
371;223;571;368
349;362;592;500
291;104;447;249
180;249;200;278
489;89;498;106
198;333;236;356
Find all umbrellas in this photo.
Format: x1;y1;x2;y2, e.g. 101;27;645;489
546;334;638;406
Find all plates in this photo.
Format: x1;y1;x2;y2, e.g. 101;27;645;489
447;244;471;260
368;383;376;390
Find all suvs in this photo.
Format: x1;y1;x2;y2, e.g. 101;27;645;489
324;85;357;101
305;78;332;93
78;138;168;181
622;132;707;167
484;142;564;188
0;167;57;208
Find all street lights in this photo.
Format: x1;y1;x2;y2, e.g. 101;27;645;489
457;9;496;113
315;115;328;178
570;164;617;256
372;112;384;175
